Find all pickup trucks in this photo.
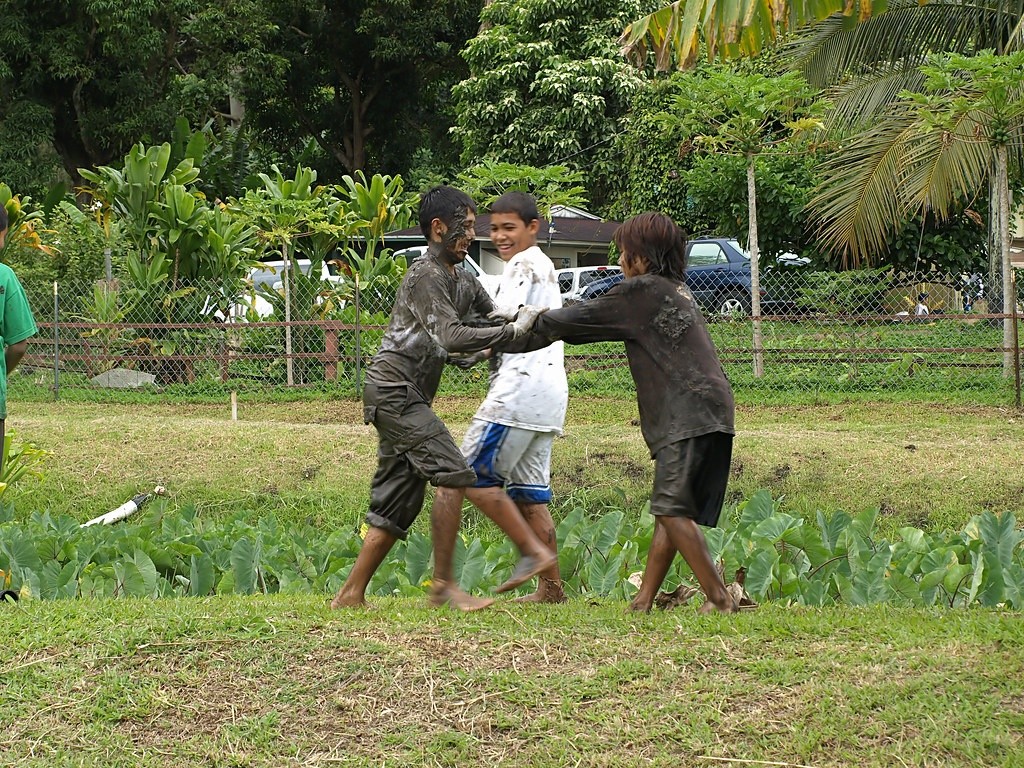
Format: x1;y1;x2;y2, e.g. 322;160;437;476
392;246;505;307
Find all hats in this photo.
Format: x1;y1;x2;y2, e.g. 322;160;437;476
915;293;929;302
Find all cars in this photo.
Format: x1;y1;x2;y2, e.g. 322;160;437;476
555;264;622;305
578;235;816;321
200;259;351;323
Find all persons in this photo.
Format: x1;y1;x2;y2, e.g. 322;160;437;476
915;292;929;323
454;191;569;605
0;203;40;467
329;185;551;612
486;210;738;617
